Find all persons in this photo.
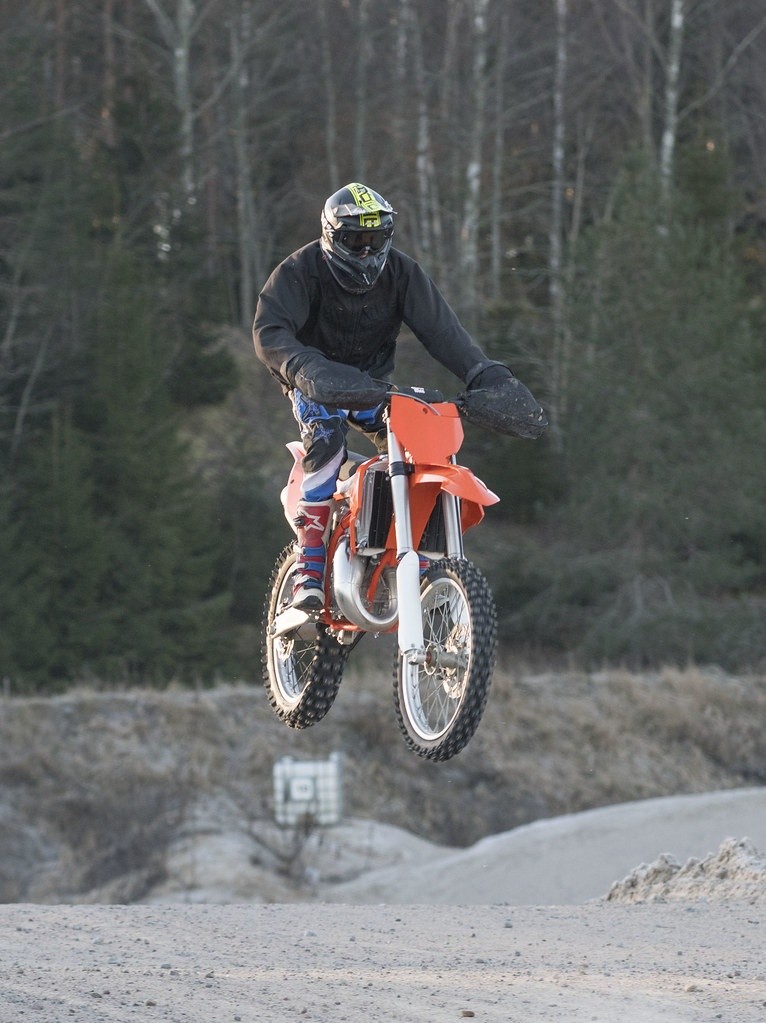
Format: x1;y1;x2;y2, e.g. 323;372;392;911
251;183;547;615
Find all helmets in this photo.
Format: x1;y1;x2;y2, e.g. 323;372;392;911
320;182;397;287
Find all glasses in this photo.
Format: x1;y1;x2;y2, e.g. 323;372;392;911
333;224;393;254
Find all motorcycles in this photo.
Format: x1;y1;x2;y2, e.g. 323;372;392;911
261;367;547;762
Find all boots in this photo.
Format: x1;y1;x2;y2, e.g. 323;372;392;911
418;553;450;615
292;498;334;609
